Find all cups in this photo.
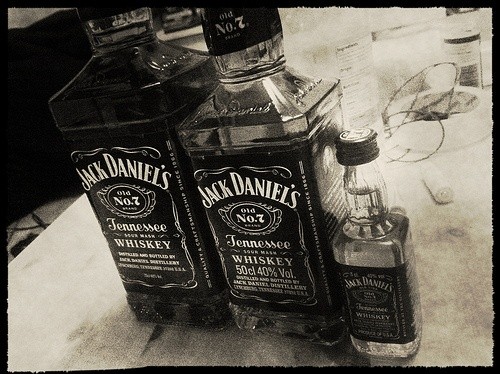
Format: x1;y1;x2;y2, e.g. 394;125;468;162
442;28;485;95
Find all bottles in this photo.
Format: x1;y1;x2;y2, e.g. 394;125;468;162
331;128;424;358
47;7;234;331
278;9;384;148
174;3;345;347
